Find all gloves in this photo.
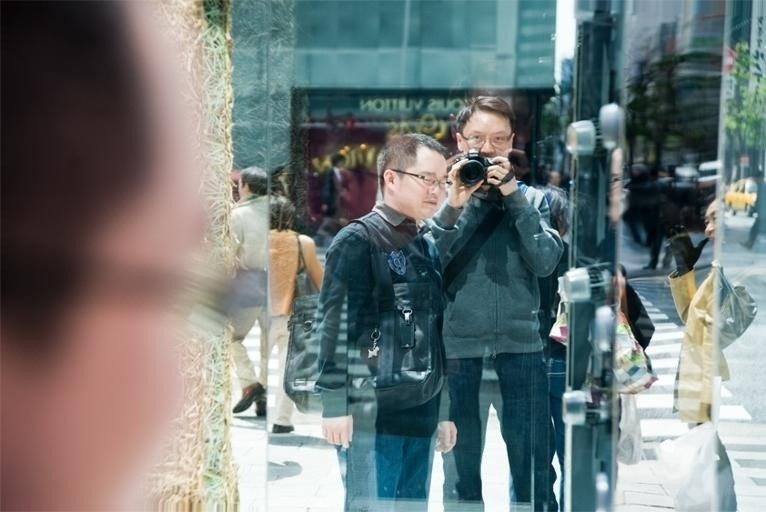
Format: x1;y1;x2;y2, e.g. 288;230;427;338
669;224;709;274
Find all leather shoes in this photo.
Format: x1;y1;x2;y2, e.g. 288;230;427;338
273;425;295;434
642;265;675;270
233;383;266;416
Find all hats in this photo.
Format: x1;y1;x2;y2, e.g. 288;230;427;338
632;163;647;175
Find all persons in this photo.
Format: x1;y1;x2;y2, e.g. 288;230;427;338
322;134;457;512
0;0;204;512
232;153;766;511
424;95;565;512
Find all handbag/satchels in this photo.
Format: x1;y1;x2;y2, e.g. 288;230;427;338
717;267;757;350
549;300;658;393
322;207;336;218
661;421;737;512
283;218;443;414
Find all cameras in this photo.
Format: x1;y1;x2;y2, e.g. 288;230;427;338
453;150;500;185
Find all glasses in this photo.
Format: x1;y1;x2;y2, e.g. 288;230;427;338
381;168;454;191
461;131;514;148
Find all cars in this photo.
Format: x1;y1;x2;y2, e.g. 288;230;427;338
724;178;757;216
